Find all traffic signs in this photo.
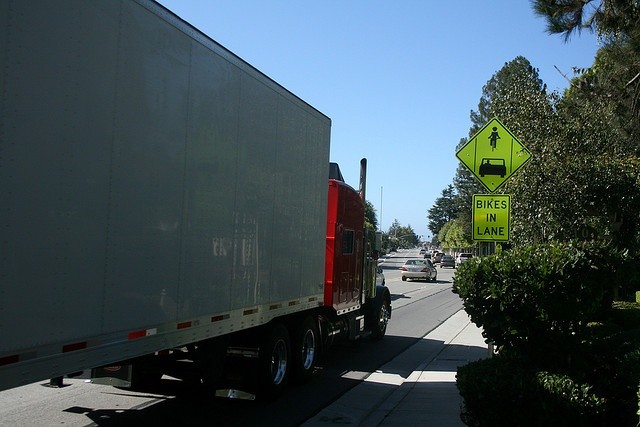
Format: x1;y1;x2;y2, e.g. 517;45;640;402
456;117;533;195
473;195;509;242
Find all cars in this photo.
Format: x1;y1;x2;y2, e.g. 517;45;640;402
420;244;444;262
441;255;455;268
457;253;473;265
402;258;436;283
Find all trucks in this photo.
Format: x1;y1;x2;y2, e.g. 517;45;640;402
0;1;392;403
